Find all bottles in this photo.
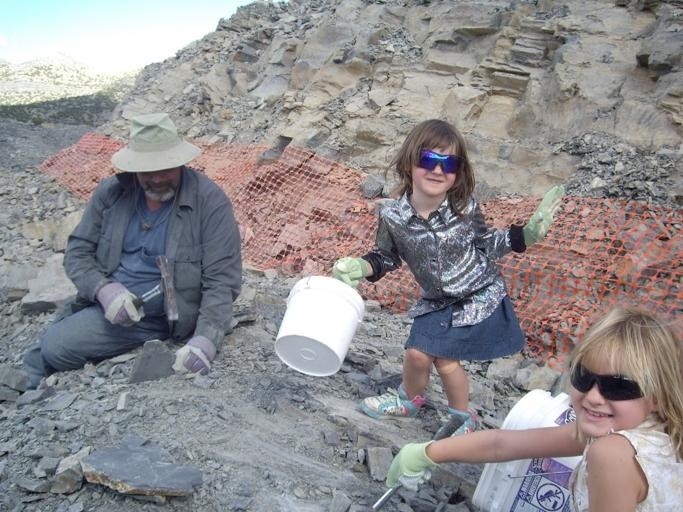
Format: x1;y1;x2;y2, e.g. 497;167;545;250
111;113;200;172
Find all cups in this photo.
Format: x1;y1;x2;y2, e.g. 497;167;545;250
569;361;644;400
413;150;460;174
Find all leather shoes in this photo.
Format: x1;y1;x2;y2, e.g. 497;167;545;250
96;282;145;328
333;257;369;288
172;336;217;376
522;185;564;247
386;440;440;492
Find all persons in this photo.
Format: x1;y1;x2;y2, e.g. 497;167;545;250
386;304;681;511
23;111;245;385
333;118;566;444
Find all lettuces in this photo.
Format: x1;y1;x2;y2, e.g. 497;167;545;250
133;255;179;321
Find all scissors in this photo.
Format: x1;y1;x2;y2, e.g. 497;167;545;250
372;414;464;508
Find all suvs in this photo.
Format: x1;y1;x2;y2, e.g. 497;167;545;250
274;275;365;377
472;388;588;512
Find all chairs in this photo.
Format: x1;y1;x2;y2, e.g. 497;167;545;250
360;385;425;422
435;407;480;437
